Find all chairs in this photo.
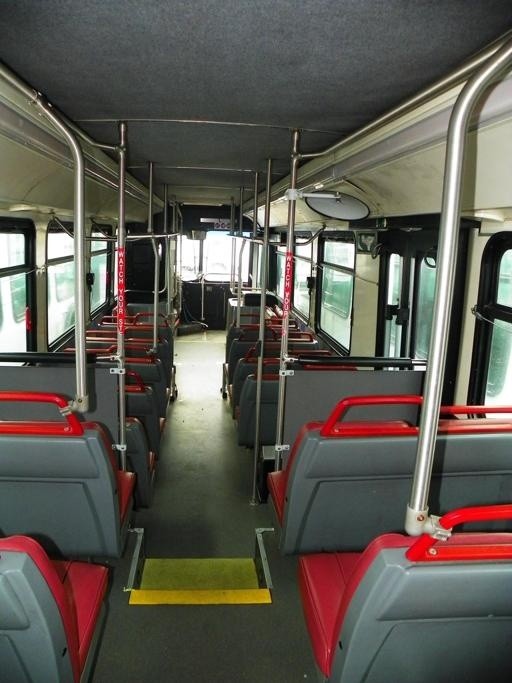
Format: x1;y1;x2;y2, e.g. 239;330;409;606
217;314;510;682
2;312;178;680
